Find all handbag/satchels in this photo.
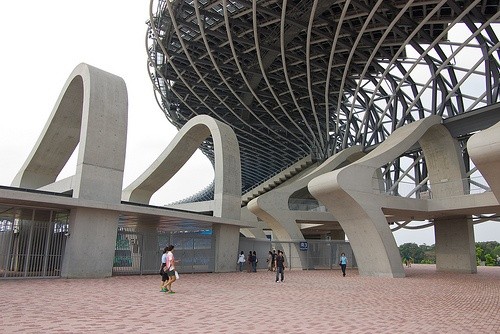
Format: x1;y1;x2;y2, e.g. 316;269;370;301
175;270;180;279
163;266;169;273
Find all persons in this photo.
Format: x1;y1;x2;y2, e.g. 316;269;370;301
160;247;169;292
340;253;348;277
268;250;286;284
247;251;252;273
239;251;245;272
162;245;176;294
252;251;257;272
